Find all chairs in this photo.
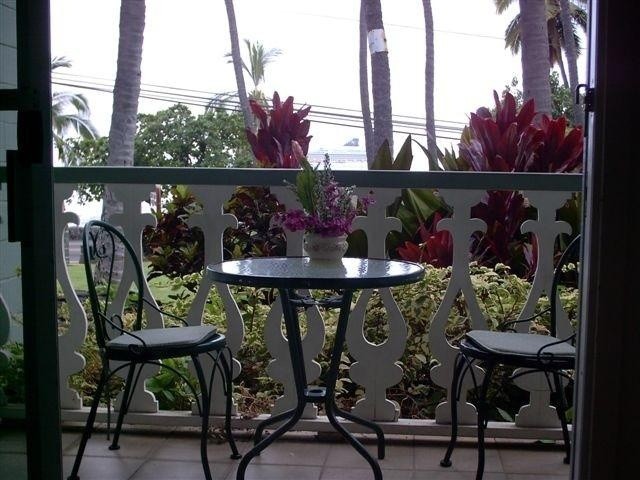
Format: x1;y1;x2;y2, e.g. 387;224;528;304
439;234;583;480
66;218;242;480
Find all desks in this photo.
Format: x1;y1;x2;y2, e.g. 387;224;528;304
206;256;426;480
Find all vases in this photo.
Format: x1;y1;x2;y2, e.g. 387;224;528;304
303;233;349;261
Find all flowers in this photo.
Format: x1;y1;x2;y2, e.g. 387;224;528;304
273;139;377;237
